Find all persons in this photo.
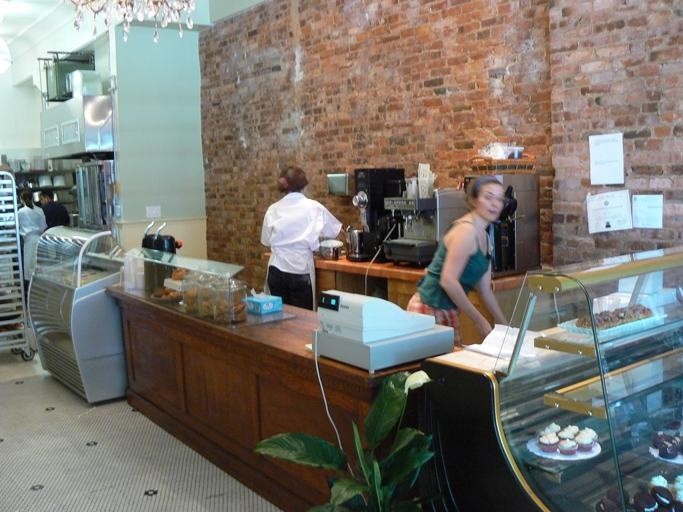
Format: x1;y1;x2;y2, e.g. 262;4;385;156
260;166;344;313
407;174;508;346
17;190;48;281
39;191;70;228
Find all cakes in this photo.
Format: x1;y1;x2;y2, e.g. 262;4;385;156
538;422;683;512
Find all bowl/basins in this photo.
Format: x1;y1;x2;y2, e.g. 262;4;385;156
317;239;344;261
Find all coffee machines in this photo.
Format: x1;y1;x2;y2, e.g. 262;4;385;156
344;167;405;261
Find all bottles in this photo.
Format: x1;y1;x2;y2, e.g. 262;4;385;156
183;269;249;323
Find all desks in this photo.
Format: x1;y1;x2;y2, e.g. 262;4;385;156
262;251;531;345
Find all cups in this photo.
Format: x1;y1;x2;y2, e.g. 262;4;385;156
37;174;67;188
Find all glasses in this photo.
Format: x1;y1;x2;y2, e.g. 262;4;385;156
478;193;510;207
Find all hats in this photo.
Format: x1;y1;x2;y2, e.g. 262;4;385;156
277;165;308;193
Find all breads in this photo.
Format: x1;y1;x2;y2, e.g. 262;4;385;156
575;303;652;331
152;268;247;323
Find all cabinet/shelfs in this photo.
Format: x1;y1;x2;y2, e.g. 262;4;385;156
412;256;683;512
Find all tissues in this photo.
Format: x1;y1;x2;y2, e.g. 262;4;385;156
241;287;283;315
